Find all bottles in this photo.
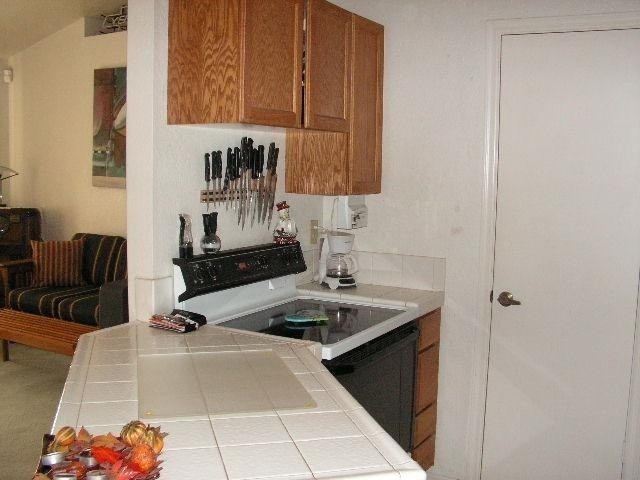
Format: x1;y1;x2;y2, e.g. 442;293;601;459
200;211;221;254
179;213;193;260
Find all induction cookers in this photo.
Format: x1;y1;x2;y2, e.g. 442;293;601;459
212;297;407;345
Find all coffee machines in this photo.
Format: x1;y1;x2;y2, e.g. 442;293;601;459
317;232;358;290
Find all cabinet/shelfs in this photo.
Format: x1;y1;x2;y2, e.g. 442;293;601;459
166;0;349;135
284;14;385;199
412;309;442;471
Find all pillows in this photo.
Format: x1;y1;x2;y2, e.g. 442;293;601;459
28;237;86;291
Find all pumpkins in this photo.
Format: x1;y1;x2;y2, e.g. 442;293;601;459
32;420;170;480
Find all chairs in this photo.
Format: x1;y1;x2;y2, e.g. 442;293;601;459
0;207;46;289
0;230;126;361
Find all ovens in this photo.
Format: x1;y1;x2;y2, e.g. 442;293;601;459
321;321;420;453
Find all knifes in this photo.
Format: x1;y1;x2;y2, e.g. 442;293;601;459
204;137;278;230
